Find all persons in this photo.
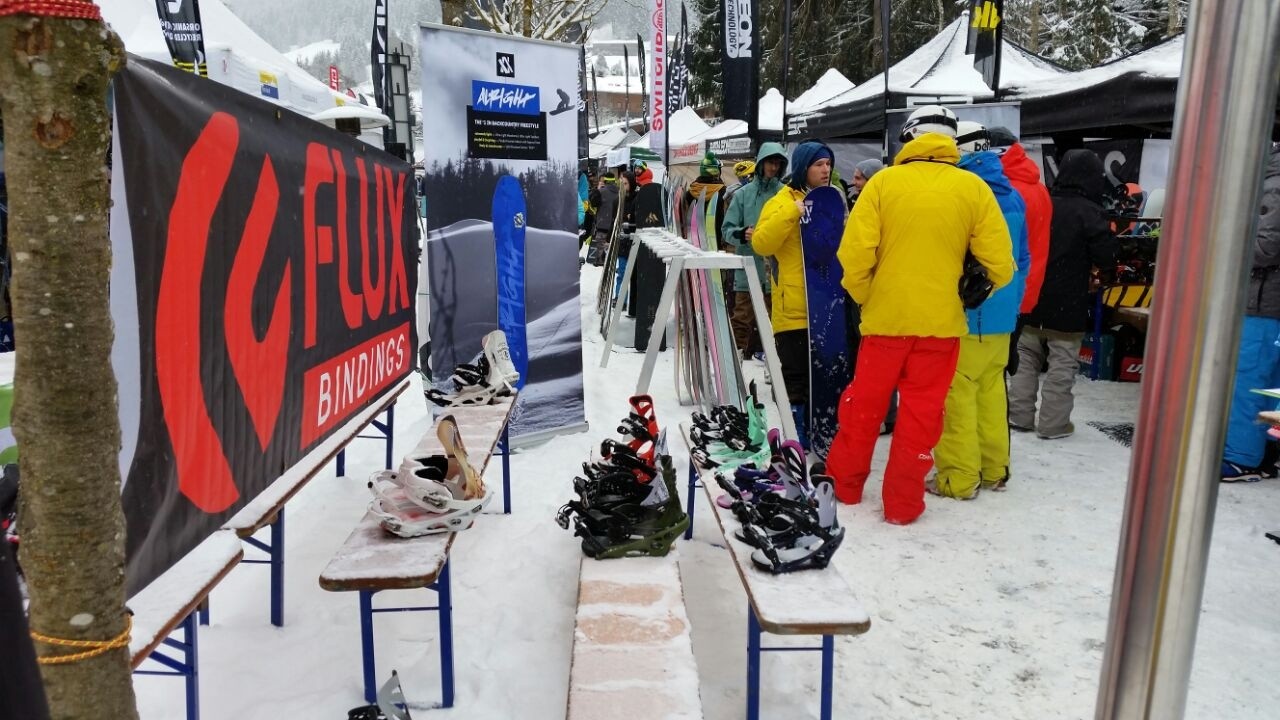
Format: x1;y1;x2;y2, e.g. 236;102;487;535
1214;141;1278;480
573;105;1118;527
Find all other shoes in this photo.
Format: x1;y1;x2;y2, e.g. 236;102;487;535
1037;422;1074;438
363;324;845;576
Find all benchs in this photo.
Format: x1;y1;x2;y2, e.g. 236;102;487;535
198;381;411;626
124;530;245;720
566;540;704;720
678;420;871;720
317;393;519;706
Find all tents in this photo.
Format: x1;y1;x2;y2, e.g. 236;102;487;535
589;8;1279;197
91;1;386;153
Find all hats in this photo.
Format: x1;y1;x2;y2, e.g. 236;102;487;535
700;152;722;178
855;158;883;180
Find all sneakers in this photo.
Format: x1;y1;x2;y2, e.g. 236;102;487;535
1220;461;1262;481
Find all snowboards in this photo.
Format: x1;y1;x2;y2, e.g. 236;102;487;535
595;172;766;432
802;184;861;464
491;172;531;391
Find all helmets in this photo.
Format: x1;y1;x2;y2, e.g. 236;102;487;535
899;104;957;142
957;120;990;157
